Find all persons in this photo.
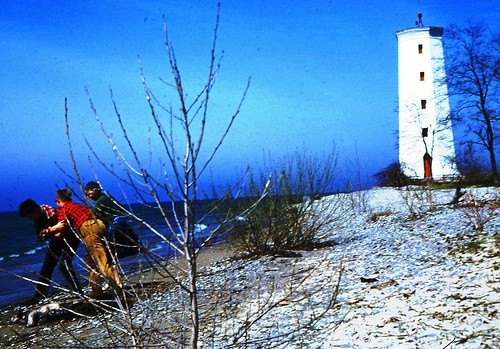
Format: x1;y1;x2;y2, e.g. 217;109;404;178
38;186;116;305
18;197;85;304
84;180;126;269
